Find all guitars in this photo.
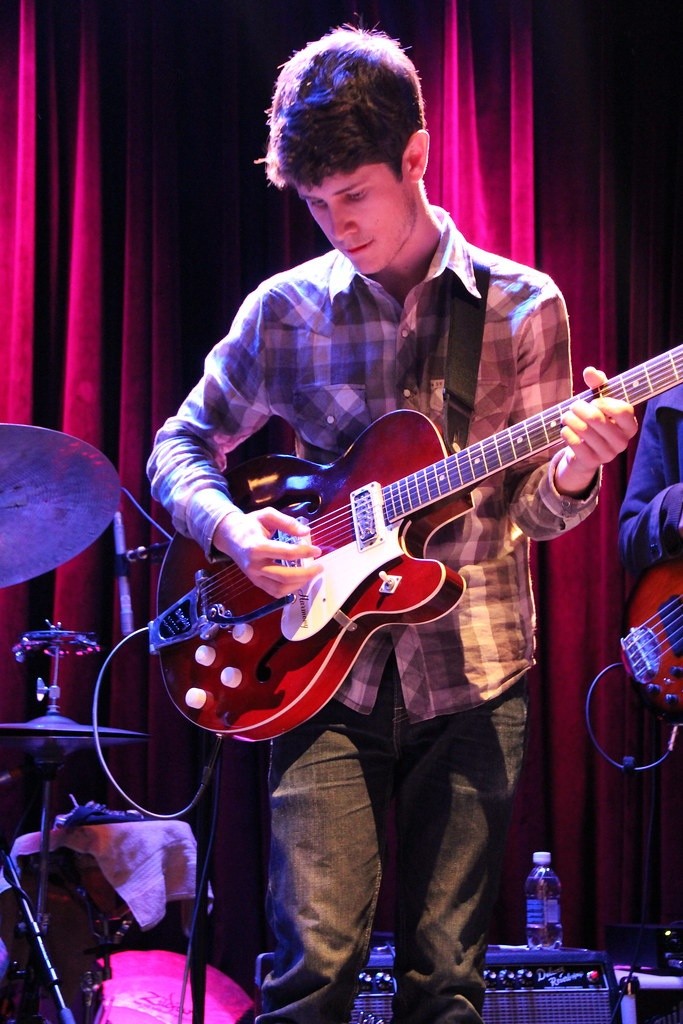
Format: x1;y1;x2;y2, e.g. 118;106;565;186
147;338;683;745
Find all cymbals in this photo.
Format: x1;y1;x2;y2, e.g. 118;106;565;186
0;422;121;594
0;713;151;764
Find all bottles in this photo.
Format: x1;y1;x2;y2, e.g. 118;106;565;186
524;851;563;952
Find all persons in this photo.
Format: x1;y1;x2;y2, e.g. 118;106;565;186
620;379;682;569
149;14;638;1024
